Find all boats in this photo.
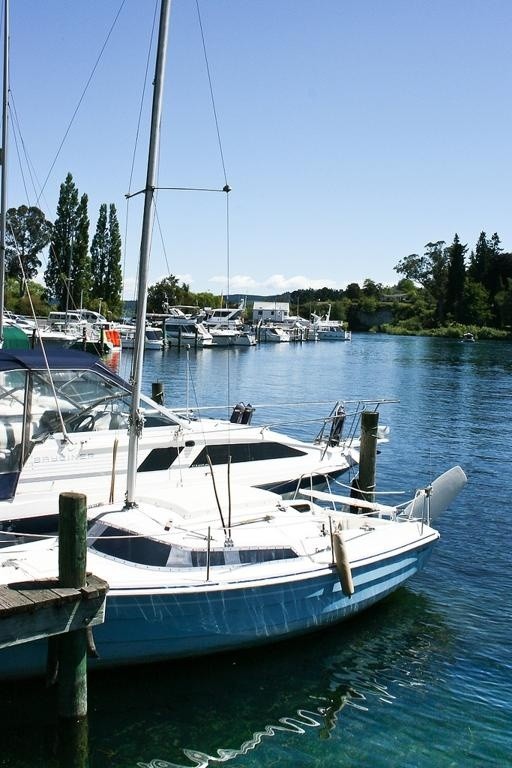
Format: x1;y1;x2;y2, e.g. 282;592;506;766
0;242;403;505
0;0;474;696
7;240;355;351
